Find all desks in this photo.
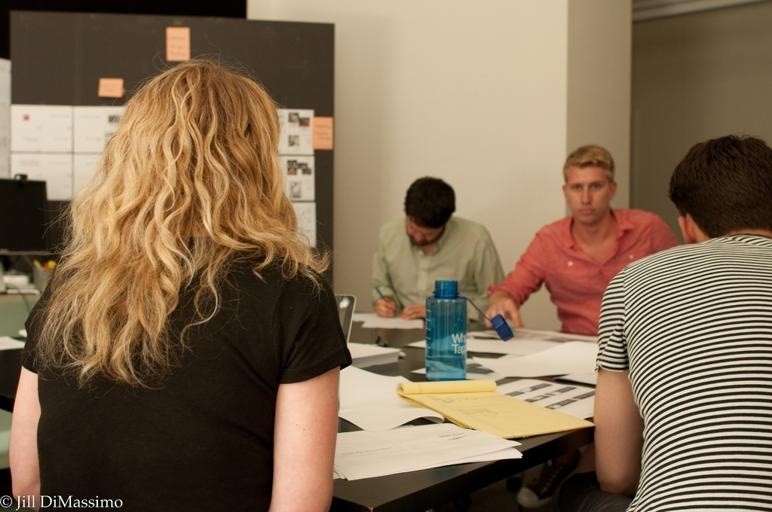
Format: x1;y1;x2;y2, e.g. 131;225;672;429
0;309;598;511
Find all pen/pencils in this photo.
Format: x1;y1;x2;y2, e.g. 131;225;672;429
375;286;386;300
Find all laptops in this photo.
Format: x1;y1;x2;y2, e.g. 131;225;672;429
334;292;356;343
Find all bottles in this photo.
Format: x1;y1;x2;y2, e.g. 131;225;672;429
426;278;468;380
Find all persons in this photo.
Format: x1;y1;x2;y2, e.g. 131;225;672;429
594;134;772;512
372;176;505;323
485;144;680;335
8;59;352;512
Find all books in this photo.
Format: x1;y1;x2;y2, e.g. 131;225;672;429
397;377;595;441
